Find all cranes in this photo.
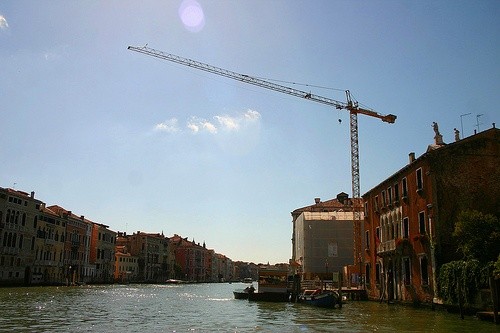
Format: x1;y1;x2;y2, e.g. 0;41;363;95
127;45;397;269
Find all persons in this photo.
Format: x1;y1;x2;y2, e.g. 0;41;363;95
362;272;367;288
314;273;322;289
357;272;361;289
243;286;251;292
250;283;256;292
294;268;302;283
431;121;440;134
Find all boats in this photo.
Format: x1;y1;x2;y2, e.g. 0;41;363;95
233;287;339;309
